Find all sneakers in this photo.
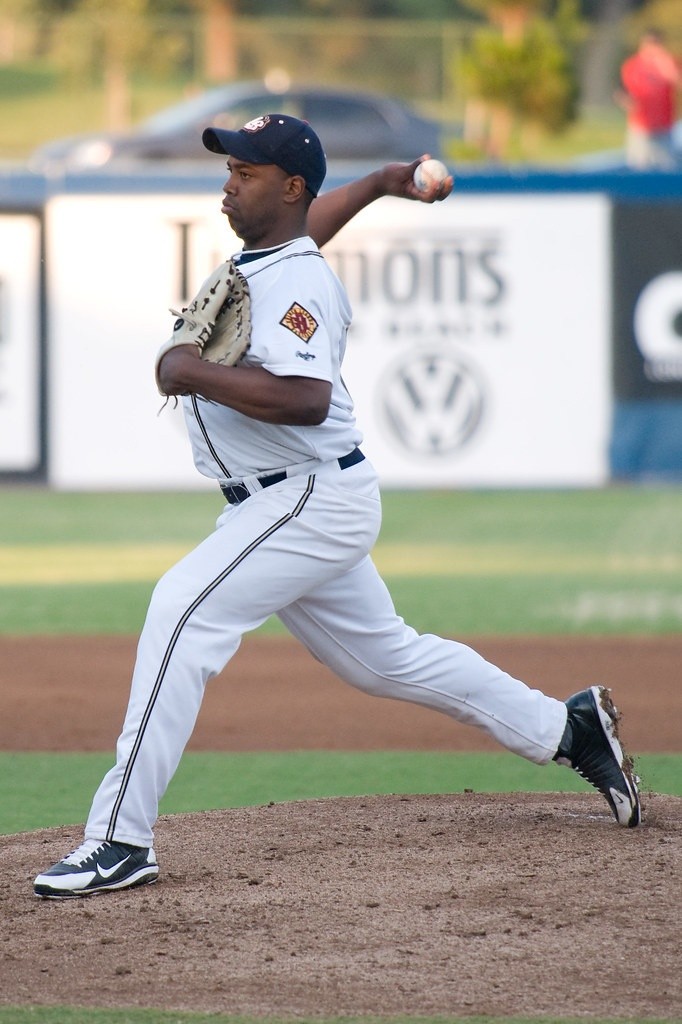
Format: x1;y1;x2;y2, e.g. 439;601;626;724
550;685;642;829
34;837;160;902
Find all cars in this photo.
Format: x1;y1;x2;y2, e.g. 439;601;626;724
34;79;496;177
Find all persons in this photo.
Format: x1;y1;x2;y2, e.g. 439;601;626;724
34;113;645;900
612;25;682;170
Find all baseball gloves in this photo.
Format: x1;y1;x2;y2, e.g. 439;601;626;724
154;259;253;400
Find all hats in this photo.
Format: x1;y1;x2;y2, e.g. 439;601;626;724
203;113;326;196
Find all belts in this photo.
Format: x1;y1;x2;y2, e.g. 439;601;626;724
220;448;365;502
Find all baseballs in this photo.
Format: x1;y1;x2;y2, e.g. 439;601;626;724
414;159;448;192
